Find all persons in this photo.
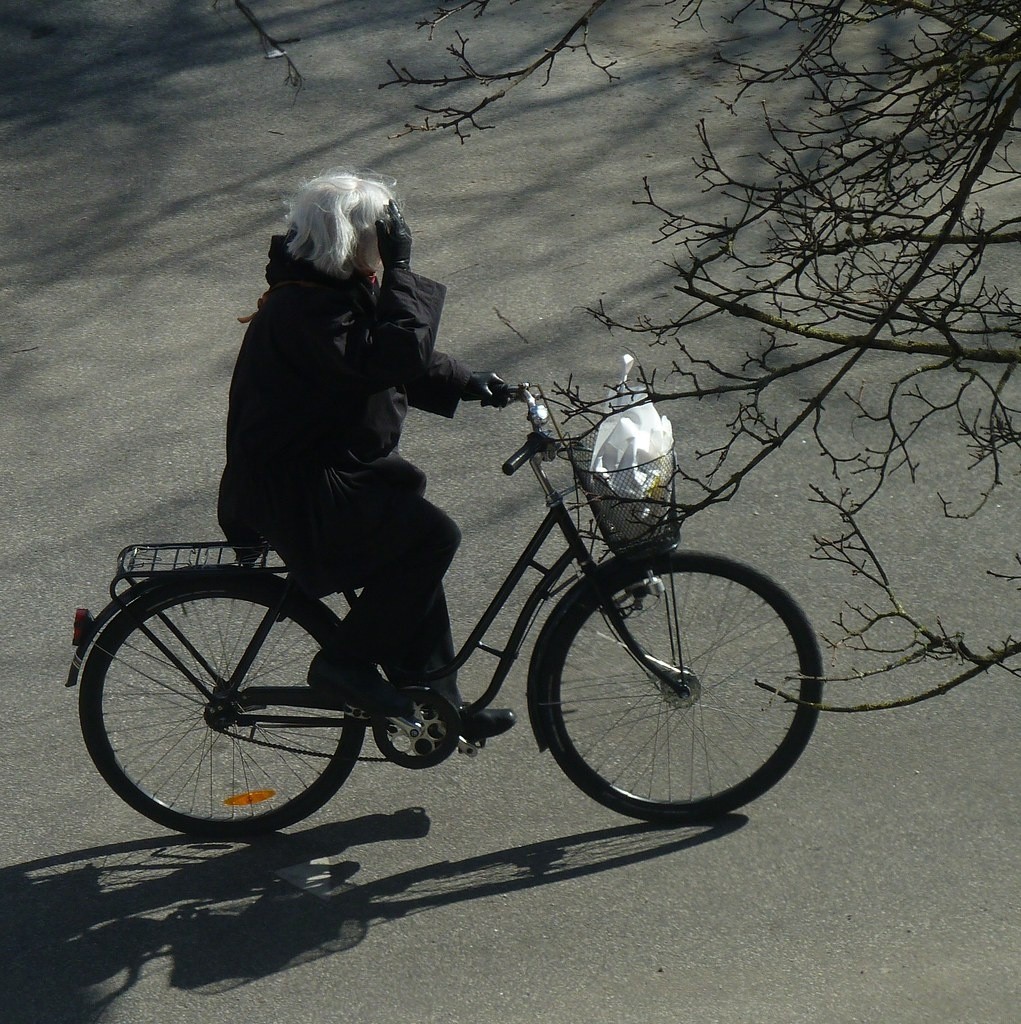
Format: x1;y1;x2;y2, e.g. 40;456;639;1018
217;162;515;740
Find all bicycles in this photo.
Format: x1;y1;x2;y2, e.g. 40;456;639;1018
65;382;825;836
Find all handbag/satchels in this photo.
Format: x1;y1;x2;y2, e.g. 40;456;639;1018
589;355;675;540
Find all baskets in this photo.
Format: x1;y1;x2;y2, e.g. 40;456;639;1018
567;425;679;563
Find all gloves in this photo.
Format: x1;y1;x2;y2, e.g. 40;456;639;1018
375;200;412;272
463;369;509;410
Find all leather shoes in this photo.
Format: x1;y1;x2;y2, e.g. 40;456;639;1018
307;651;414;718
454;702;518;737
